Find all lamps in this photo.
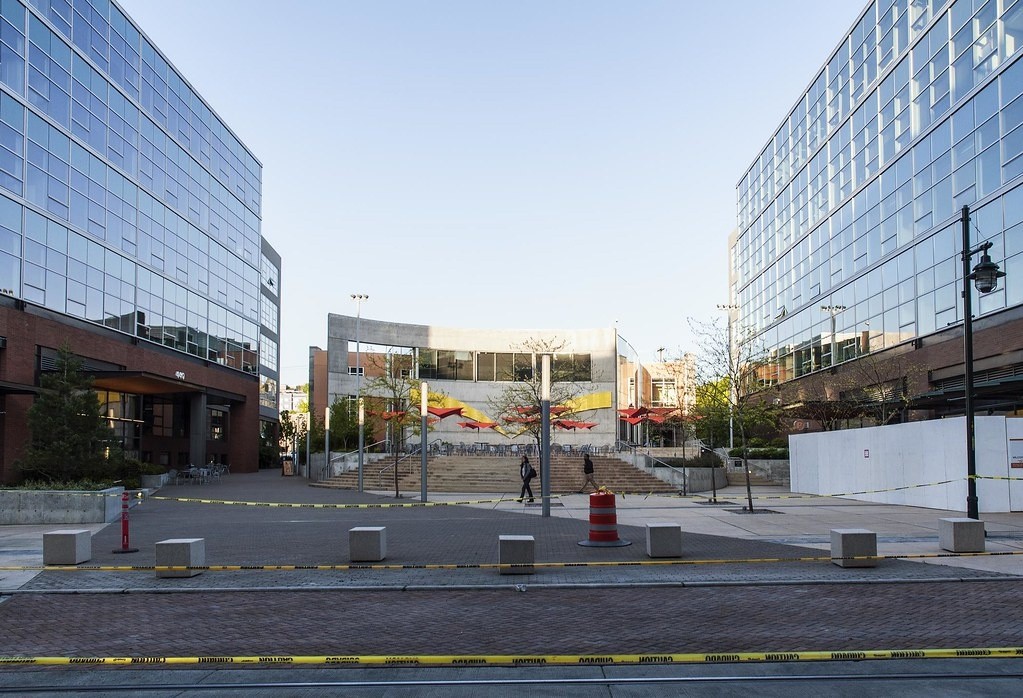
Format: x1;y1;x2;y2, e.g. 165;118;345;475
133;337;139;346
18;300;27;311
205;360;210;367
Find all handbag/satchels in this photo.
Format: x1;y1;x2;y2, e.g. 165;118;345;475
527;468;537;478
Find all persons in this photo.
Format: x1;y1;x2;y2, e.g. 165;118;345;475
576;454;598;493
517;456;534;502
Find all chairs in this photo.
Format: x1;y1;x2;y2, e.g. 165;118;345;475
404;441;615;457
168;462;231;486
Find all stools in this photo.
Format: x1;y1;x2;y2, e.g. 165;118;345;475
829;528;877;568
646;522;681;558
348;526;387;562
155;537;206;578
939;517;985;553
498;535;535;575
43;530;91;565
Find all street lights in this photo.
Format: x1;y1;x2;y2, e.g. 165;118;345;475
714;302;742;449
349;293;370;420
957;204;1006;538
820;305;848;365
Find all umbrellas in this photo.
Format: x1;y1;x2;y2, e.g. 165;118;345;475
618;406;706;444
366;410;408;420
417;405;465;455
501;406;600;444
458;420;499;441
